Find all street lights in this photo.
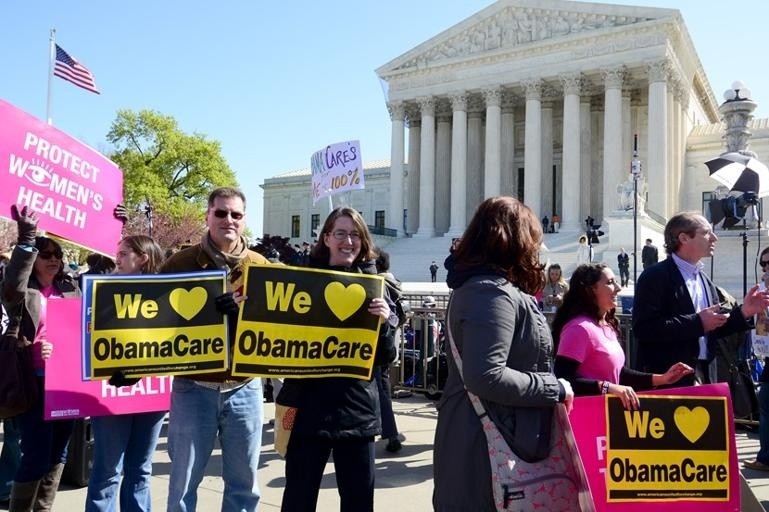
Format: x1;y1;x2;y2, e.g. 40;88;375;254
145;204;155;238
630;133;641;284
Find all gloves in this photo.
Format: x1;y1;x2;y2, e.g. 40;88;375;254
12;205;40;247
115;204;127;218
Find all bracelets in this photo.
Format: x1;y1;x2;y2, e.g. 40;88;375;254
600;380;609;394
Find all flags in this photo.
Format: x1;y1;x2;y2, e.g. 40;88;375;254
51;43;99;94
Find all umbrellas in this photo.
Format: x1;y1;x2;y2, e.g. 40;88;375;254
703;151;769;197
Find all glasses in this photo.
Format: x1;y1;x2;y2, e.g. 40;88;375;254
328;230;361;241
210;208;246;219
760;260;769;267
40;251;63;259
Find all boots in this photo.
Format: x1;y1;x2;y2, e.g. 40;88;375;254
33;462;65;512
9;480;40;512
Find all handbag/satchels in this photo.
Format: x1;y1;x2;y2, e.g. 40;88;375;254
0;332;38;418
716;353;753;418
480;406;582;512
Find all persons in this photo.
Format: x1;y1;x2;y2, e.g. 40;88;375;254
3;188;407;511
433;197;574;511
430;260;439;282
537;212;659;326
632;210;768;389
742;246;768;471
551;263;694;410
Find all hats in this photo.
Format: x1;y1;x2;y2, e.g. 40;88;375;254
424;296;437;306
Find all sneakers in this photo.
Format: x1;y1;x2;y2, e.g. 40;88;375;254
744;459;769;471
386;441;402;451
